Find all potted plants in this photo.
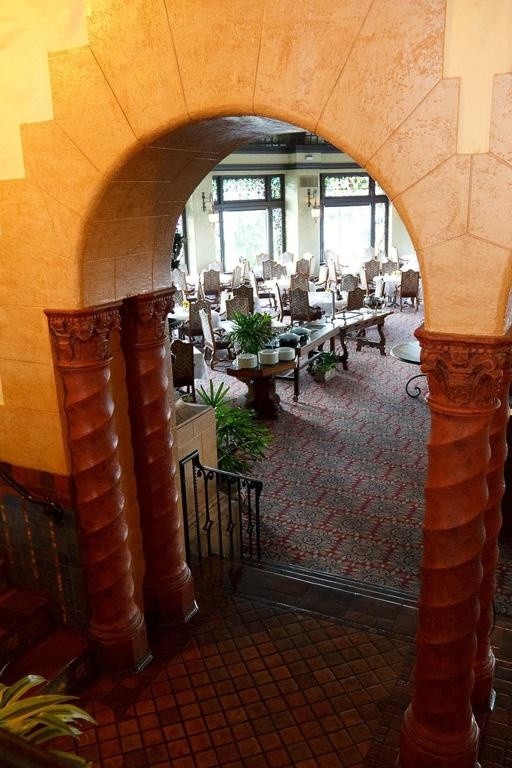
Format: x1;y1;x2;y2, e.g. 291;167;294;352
312;351;338;383
195;378;275;501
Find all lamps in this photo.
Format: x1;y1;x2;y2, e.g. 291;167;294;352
202;192;219;230
307;188;320;225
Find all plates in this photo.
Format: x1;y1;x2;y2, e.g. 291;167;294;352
238;346;295;369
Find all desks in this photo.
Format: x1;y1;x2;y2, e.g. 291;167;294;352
223;320;340;421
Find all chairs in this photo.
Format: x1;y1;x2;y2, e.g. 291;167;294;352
172;251;419;372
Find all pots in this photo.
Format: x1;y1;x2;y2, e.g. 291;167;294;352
278;326;311;348
